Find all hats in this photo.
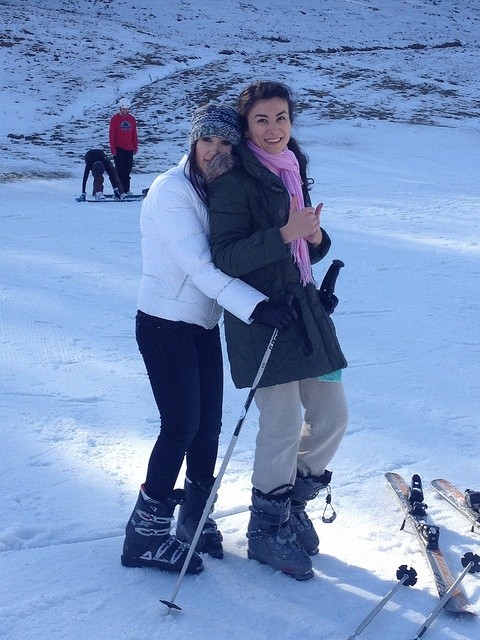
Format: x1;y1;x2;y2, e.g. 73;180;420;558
189;102;243;147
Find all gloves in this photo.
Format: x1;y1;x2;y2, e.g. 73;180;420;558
252;300;299;331
80;193;86;199
114;187;122;200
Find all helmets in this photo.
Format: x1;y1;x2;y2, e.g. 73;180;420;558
118;98;131;108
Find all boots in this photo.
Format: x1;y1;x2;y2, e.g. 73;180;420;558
177;475;223;555
247;487;313;581
123;484;201;566
290;471;337;555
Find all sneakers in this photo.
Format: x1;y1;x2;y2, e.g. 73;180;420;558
95;192;105;200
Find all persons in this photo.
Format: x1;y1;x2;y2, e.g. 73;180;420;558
79;146;130;203
113;98;340;578
109;98;138;196
208;81;351;581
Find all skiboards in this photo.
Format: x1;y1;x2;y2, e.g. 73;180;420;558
75;195;146;203
104;195;145;198
384;472;480;616
